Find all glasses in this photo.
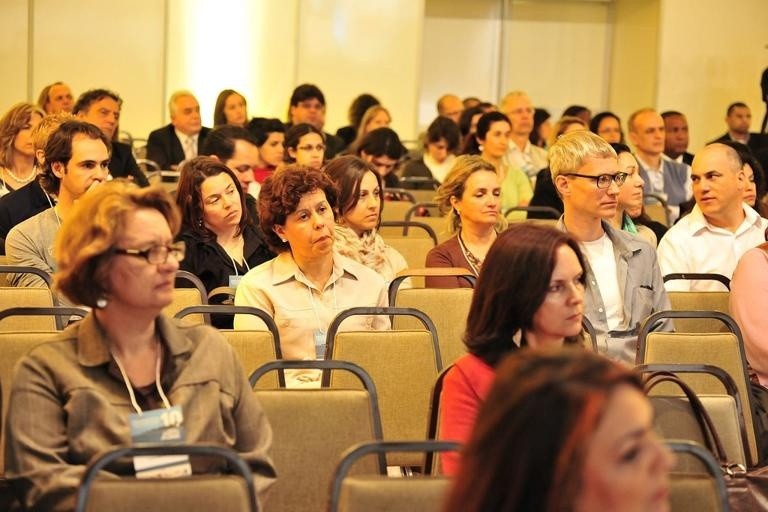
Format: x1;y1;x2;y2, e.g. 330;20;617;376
563;173;628;188
302;144;327;151
111;240;186;265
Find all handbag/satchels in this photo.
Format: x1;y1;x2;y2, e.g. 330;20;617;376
718;462;768;512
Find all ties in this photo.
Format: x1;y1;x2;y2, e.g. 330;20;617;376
185;137;194;165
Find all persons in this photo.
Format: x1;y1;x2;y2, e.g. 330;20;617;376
404;90;767;462
1;176;278;511
1;81;151;287
146;83;403;327
546;127;677;371
233;160;393;392
439;346;677;512
437;220;589;483
